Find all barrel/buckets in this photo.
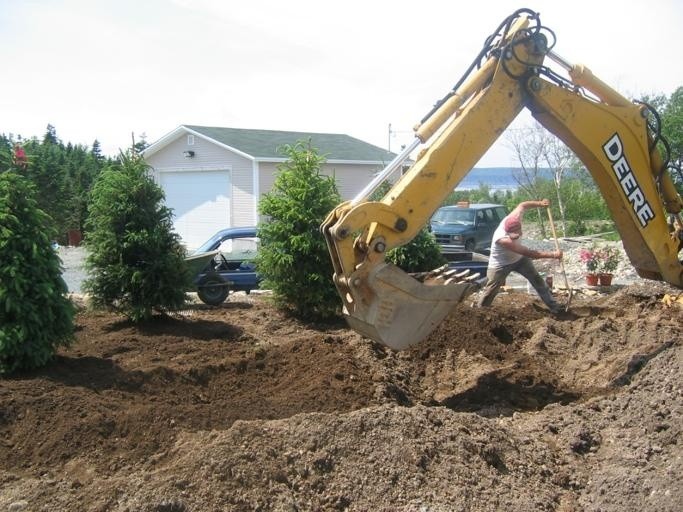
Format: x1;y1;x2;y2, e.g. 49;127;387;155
527;272;547;297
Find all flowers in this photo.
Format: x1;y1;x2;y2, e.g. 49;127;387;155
580;240;620;273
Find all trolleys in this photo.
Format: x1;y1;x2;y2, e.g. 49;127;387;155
181;250;236;305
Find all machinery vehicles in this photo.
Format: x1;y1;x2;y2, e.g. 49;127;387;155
319;9;681;348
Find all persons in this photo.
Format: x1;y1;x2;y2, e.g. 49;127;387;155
667;216;676;232
477;197;564;315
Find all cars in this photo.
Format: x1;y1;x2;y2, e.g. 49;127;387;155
191;228;269;290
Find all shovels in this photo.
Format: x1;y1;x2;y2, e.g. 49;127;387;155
546;207;573;313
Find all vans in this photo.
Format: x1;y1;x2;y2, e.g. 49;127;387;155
428;199;510;254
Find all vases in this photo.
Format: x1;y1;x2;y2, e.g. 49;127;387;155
584;274;613;287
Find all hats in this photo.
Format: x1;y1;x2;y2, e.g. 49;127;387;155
505;216;522;240
